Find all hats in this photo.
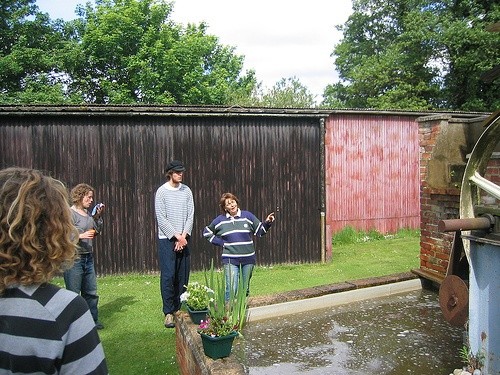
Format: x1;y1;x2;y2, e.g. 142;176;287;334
165;160;185;171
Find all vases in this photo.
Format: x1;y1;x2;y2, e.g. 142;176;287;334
187;306;209;324
200;331;239;359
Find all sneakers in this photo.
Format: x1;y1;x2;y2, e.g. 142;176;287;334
165;314;175;328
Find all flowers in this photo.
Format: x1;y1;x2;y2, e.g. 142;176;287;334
180;281;214;310
197;318;235;337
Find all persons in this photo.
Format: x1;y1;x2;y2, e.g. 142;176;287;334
0;167;110;375
202;193;275;301
63;183;104;329
154;160;195;327
